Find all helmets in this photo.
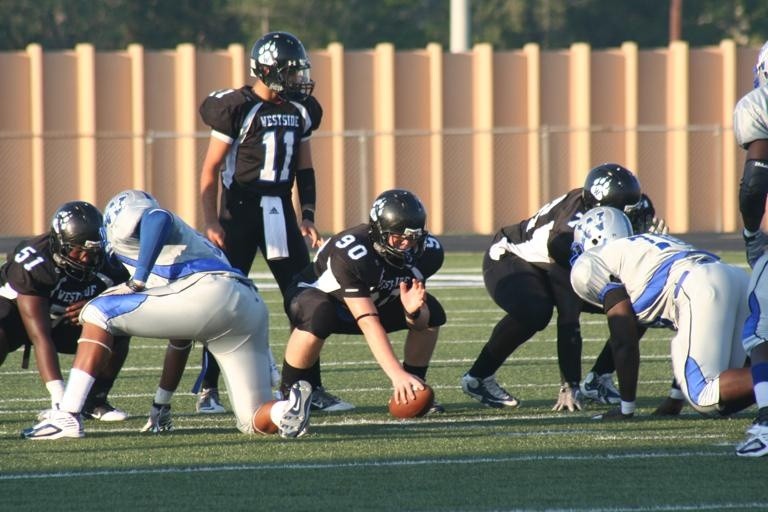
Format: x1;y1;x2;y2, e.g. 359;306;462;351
752;40;768;90
367;190;429;273
567;163;653;266
250;33;314;102
50;189;159;282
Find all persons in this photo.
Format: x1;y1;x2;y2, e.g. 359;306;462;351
282;188;448;415
463;163;656;413
192;31;357;416
569;205;767;421
1;201;134;422
732;42;768;458
19;189;314;442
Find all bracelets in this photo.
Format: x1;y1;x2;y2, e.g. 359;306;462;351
125;278;146;293
403;307;420;320
302;210;313;222
665;386;688;404
559;383;579;394
618;399;636;418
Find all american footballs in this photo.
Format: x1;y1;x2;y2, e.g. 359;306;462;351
389;385;435;417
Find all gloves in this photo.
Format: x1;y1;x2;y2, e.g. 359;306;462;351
100;280;145;297
743;228;768;268
